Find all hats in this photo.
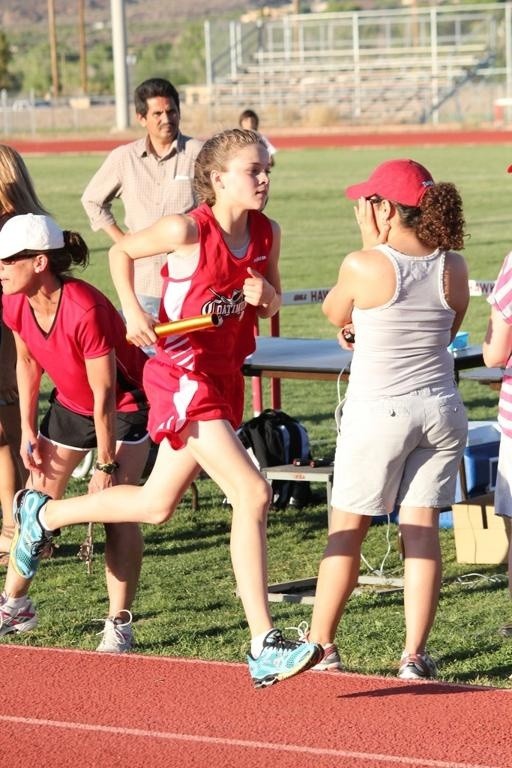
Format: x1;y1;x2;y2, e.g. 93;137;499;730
346;160;434;210
0;213;64;260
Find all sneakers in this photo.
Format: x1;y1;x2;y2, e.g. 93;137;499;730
91;609;135;653
0;593;36;638
397;648;436;681
10;490;60;580
246;621;342;689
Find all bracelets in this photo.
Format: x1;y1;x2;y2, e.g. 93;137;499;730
95;462;119;474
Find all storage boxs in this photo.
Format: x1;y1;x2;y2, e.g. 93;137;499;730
394;419;499;529
453;491;511;564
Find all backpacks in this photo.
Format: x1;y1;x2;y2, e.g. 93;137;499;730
236;409;312;508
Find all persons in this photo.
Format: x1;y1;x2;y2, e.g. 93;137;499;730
79;78;207;362
0;212;151;654
298;156;470;678
238;109;276;171
0;143;54;565
12;130;324;691
480;252;512;639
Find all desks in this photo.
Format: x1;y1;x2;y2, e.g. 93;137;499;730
241;337;488;529
